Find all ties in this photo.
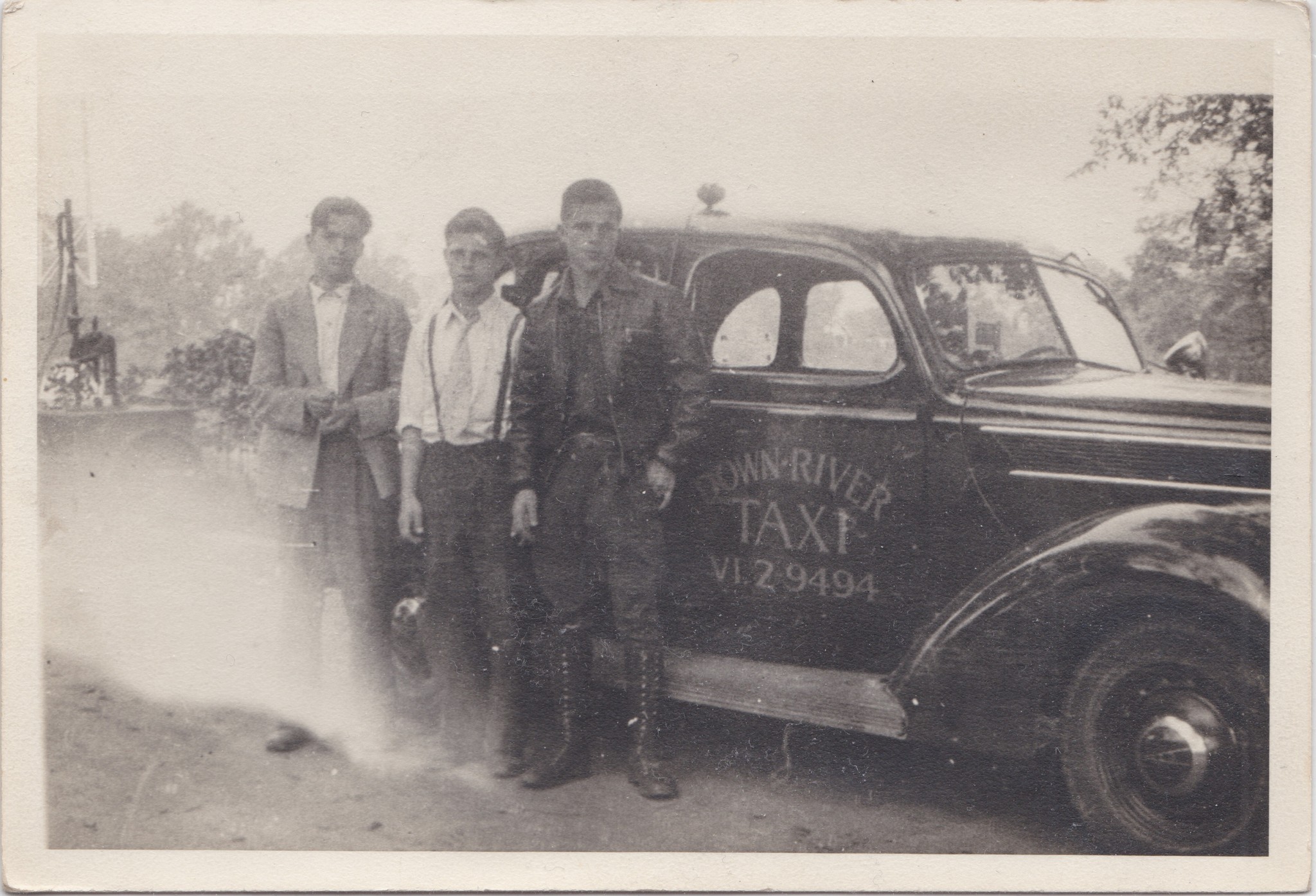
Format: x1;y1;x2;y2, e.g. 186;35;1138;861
439;315;481;441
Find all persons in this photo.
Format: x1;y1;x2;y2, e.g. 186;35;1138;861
246;196;415;751
389;209;537;778
502;173;709;800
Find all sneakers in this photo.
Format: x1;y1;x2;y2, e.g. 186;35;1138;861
265;722;313;752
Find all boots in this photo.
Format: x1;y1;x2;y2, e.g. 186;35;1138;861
519;626;594;790
485;644;524;778
624;640;679;798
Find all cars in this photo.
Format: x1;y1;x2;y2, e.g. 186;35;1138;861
387;218;1272;860
